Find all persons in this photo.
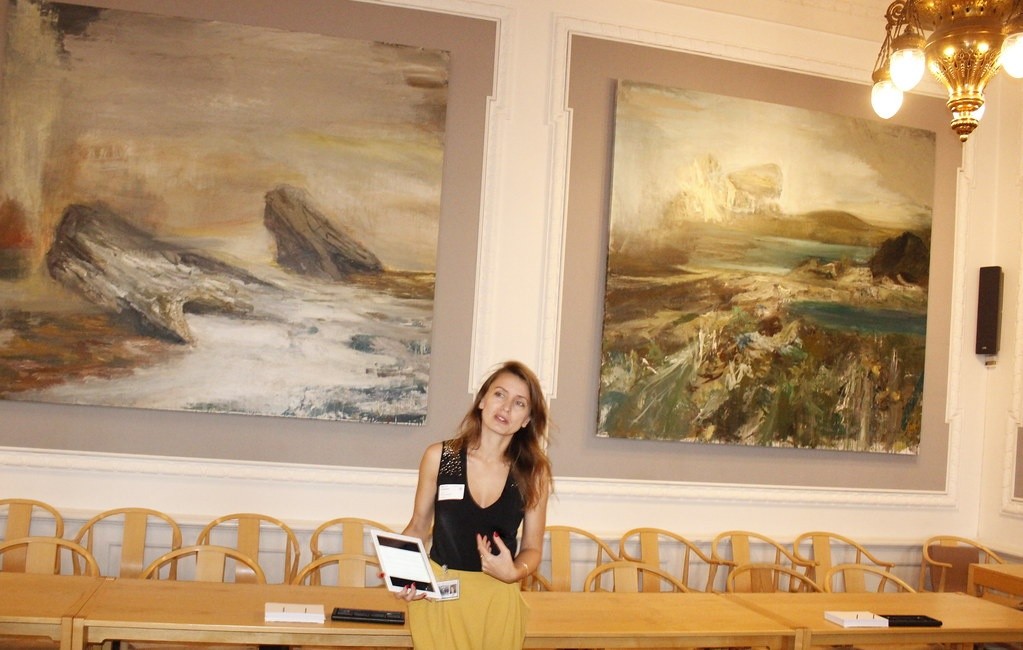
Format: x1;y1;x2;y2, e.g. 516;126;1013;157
449;585;456;594
379;360;555;602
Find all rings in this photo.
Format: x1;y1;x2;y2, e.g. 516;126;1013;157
480;555;483;558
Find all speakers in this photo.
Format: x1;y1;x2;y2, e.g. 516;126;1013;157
975;265;1002;355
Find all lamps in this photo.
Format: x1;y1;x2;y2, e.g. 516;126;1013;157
870;0;1023;143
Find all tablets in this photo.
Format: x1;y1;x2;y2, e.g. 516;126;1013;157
371;530;442;598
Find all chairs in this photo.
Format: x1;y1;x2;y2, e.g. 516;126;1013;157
0;497;1023;650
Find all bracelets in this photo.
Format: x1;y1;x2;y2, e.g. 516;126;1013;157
520;562;530;575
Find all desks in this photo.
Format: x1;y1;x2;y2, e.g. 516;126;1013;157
518;580;797;650
70;576;418;650
966;562;1023;613
720;591;1023;650
0;572;104;650
